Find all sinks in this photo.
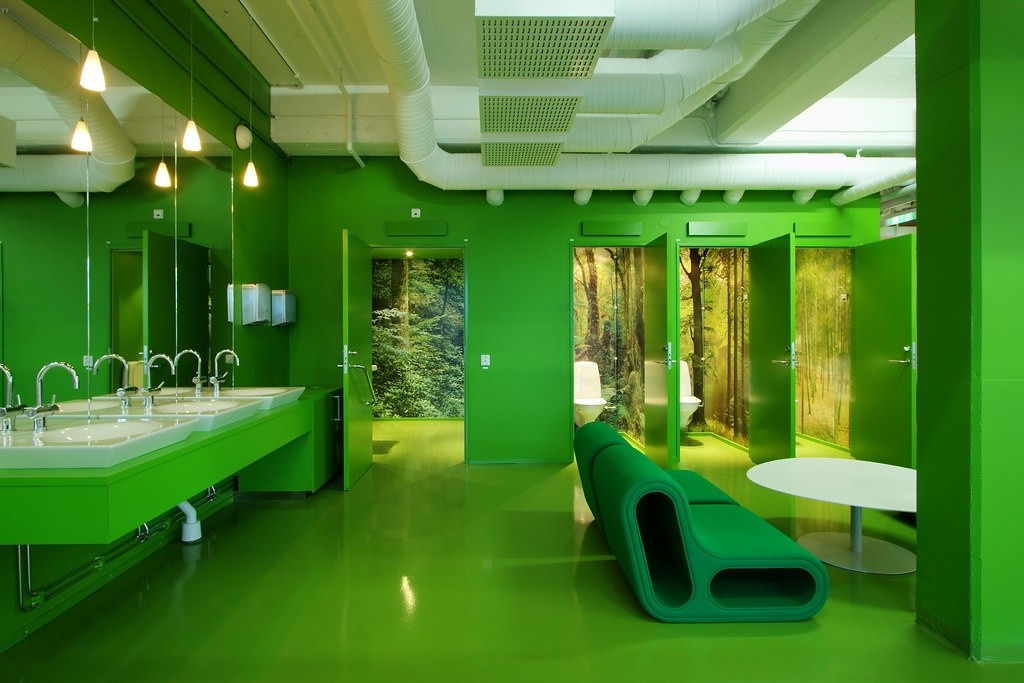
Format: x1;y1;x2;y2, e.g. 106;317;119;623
183;386;306;411
90;386;195;400
98;398;264;432
31;398;135;417
0;418;200;469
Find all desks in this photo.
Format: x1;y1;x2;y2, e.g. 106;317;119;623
745;456;917;575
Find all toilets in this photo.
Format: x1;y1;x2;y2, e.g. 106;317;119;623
680;360;701;432
574;361;607;427
645;359;667;427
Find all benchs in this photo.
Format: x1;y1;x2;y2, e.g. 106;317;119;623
594;445;830;623
572;421;741;543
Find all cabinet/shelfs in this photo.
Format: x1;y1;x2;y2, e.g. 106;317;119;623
234;383;343;496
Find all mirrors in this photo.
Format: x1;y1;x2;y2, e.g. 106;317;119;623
0;0;237;440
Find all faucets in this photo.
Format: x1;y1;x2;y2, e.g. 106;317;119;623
173;349;208;393
93;354;139;409
0;362;28;432
23;361;80;432
209;349;240;397
140;354;175;406
